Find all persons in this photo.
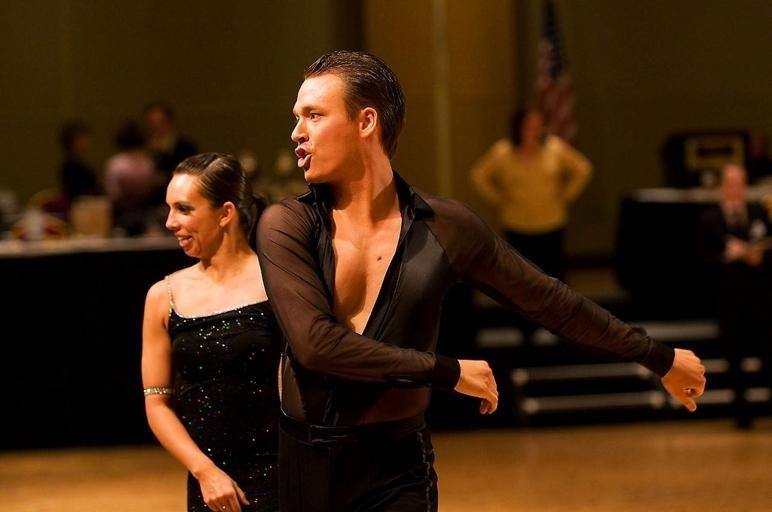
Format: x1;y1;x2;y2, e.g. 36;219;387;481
470;104;593;335
256;50;706;512
57;100;207;237
140;152;278;512
700;162;770;431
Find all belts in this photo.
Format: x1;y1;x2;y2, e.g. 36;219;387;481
279;408;425;444
